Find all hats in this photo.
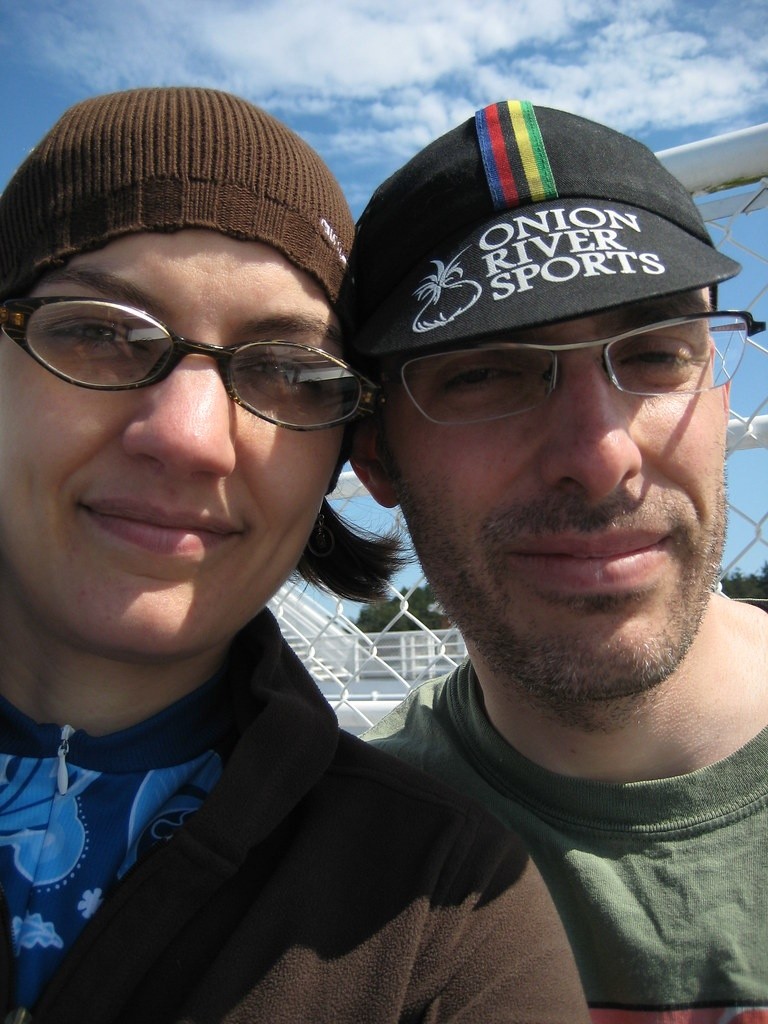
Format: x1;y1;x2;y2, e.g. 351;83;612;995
335;101;746;378
1;87;353;309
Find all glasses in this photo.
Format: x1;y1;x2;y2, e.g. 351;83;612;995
0;296;387;431
380;310;766;427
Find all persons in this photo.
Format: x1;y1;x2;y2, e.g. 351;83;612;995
326;95;768;1024
3;87;594;1023
